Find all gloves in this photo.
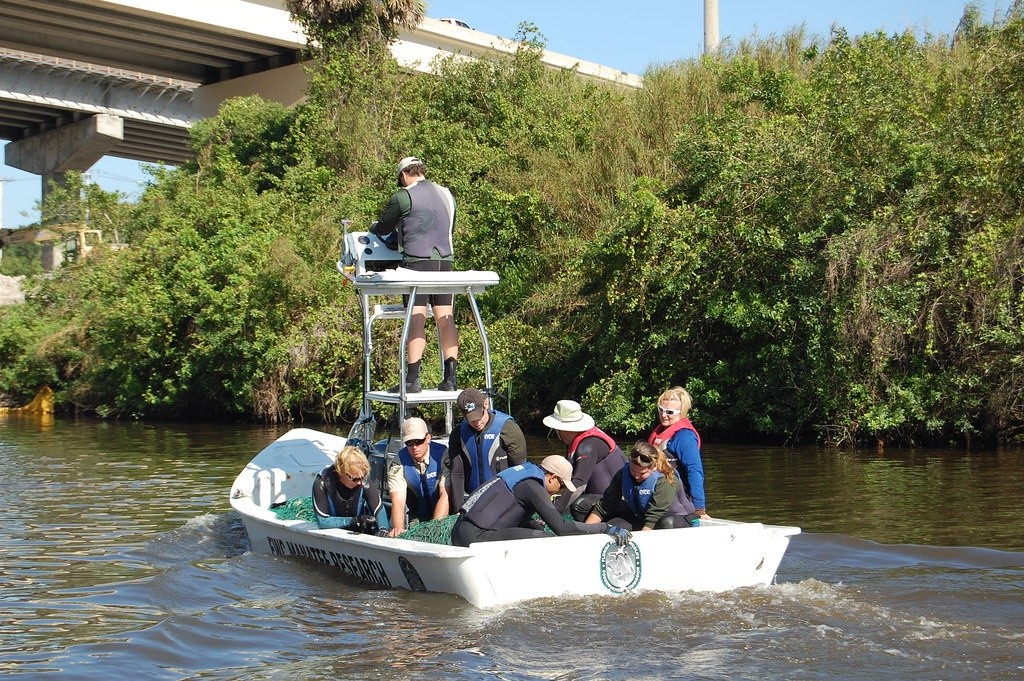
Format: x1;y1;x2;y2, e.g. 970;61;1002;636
351;515;379;535
375;527;389;538
605;523;633;547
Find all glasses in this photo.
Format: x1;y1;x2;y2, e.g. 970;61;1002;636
344;471;365;482
657;406;682;416
630;449;651;464
405;432;428;446
556;477;566;489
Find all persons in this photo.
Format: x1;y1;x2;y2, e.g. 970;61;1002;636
448;389;527;515
543;400;629;523
586;439;700;531
312;446;389;538
450;455;632;549
388;417;452;538
649;386;711;520
370;158;457;393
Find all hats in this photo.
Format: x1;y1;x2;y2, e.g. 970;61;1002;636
543;400;594;432
541;454;577;493
396;157;423;187
457;388;485;423
402;417;427;443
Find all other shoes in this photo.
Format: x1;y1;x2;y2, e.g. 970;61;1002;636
437;375;457;391
387;377;422;393
